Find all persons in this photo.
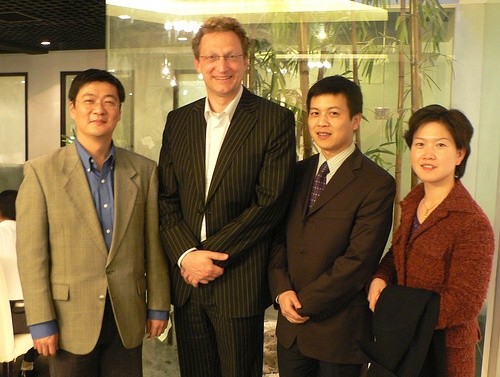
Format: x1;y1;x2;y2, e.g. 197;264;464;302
366;104;495;376
15;68;171;377
158;15;296;377
263;76;397;377
1;189;40;377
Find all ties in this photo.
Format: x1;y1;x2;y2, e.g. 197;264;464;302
306;161;331;214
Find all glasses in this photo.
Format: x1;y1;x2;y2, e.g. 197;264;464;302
197;51;247;63
71;98;120;110
